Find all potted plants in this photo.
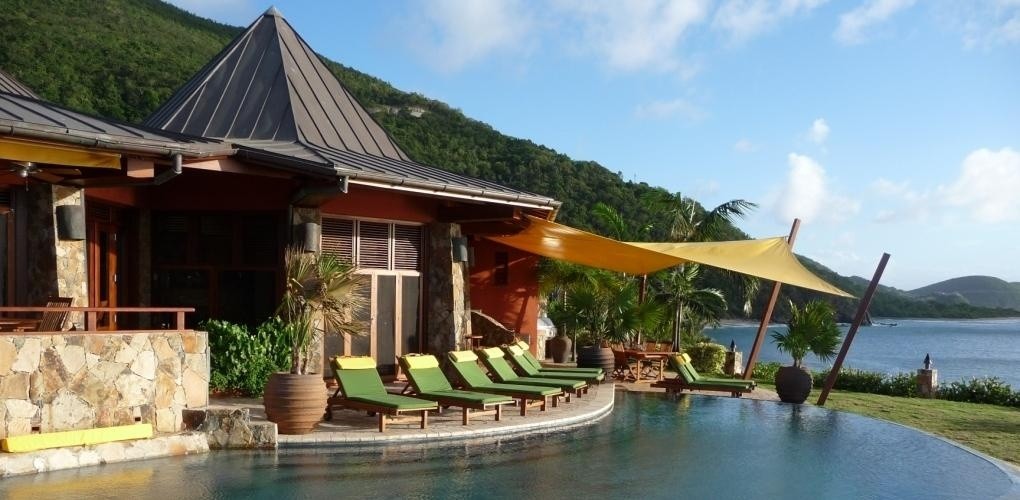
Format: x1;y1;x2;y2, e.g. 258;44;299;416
773;302;839;403
263;244;369;434
541;251;612;383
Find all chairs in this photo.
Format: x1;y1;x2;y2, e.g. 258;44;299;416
612;340;674;383
652;352;755;397
14;295;76;332
324;340;607;432
